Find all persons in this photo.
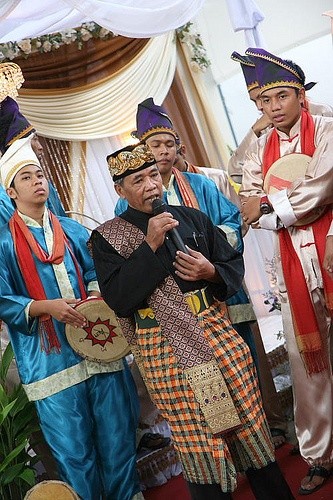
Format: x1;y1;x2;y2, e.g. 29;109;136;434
116;47;333;491
90;141;295;500
0;97;141;500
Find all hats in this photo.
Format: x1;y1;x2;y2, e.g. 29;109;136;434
0;133;42;191
0;96;37;155
231;51;260;93
136;98;179;146
106;140;156;182
245;47;304;95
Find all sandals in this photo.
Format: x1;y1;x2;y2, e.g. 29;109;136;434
271;428;286;448
298;466;329;495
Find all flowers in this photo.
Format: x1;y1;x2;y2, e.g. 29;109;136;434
0;20;212;73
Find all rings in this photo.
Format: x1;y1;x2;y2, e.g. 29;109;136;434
242;216;247;221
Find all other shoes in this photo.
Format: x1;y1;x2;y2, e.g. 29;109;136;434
136;432;171;453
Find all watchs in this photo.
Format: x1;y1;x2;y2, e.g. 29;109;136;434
261;196;271;214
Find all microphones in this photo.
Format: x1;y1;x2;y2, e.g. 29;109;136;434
152;198;191;257
